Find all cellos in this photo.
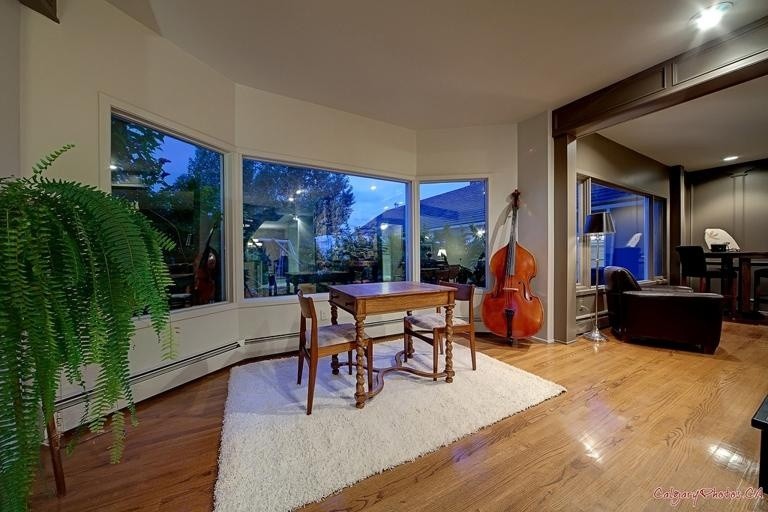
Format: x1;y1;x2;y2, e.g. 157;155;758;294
194;215;218;303
481;189;544;345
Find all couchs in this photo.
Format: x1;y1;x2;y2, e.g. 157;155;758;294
603;264;727;354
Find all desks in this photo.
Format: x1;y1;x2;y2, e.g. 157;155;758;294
284;270;358;295
703;251;768;315
328;281;459;408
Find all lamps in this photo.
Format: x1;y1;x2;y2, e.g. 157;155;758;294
581;212;616;343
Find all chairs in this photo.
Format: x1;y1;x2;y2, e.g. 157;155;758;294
674;246;737;319
296;289;374;415
403;281;478;384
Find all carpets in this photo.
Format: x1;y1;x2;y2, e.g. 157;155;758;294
210;334;567;511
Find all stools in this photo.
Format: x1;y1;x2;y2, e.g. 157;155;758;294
754;268;768;312
